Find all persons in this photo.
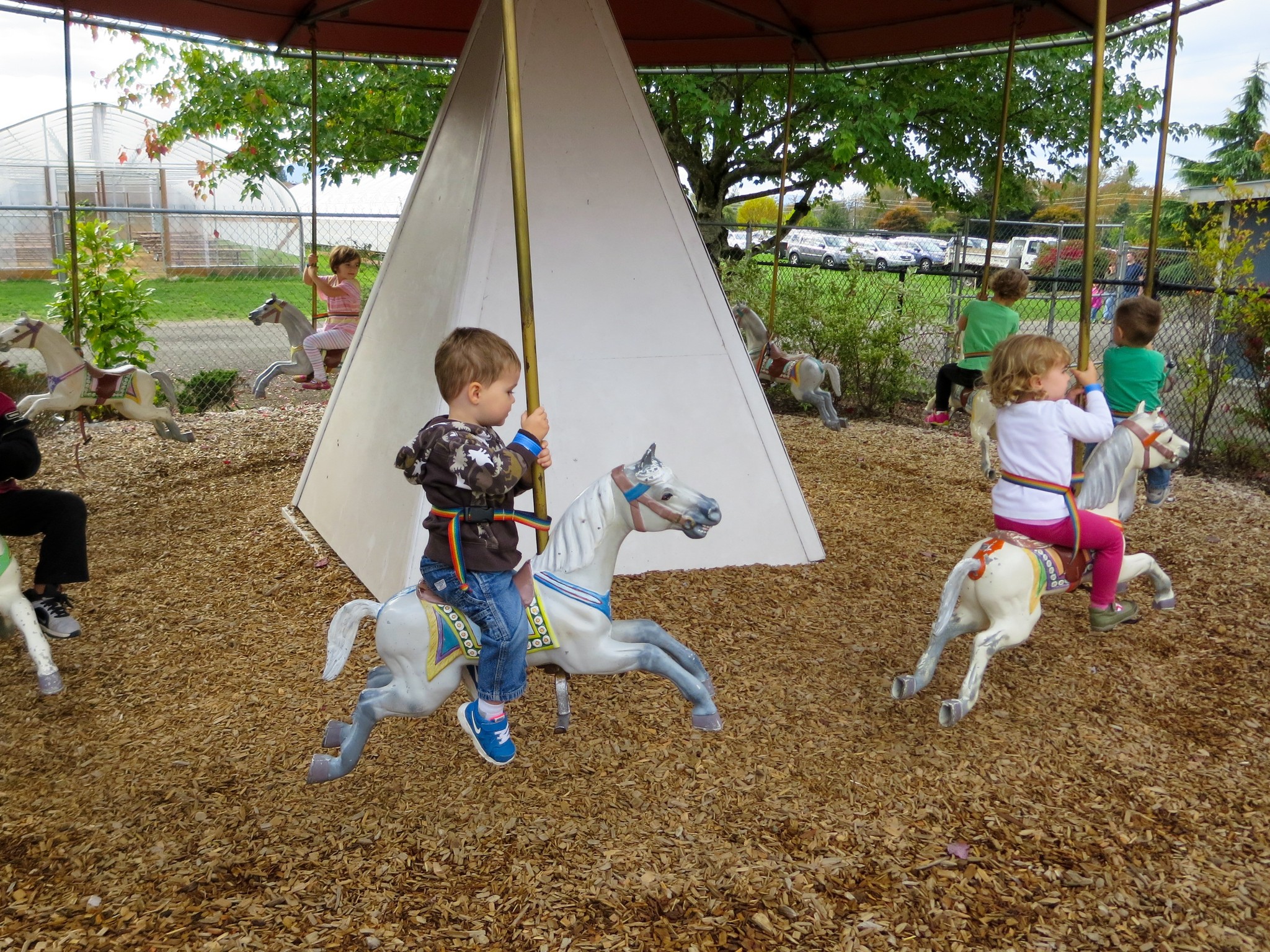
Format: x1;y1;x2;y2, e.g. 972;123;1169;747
924;267;1029;427
1121;251;1144;300
1096;261;1118;324
975;335;1140;632
0;392;90;638
293;245;361;390
1082;294;1172;508
1090;279;1104;324
394;328;554;765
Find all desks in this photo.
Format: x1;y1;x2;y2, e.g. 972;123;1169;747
133;231;253;266
0;232;71;263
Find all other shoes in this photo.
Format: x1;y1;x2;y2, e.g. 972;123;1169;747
1088;599;1138;632
1146;486;1170;509
302;379;331;390
1101;320;1106;324
293;374;308;383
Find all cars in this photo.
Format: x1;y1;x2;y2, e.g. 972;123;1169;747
727;228;1069;274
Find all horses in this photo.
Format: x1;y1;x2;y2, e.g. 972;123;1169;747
890;400;1191;727
732;297;849;433
306;443;722;784
0;535;65;694
247;292;348;398
920;323;998;482
0;311;195;443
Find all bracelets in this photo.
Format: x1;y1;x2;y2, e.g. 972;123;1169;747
1083;383;1102;393
512;434;543;457
304;263;316;268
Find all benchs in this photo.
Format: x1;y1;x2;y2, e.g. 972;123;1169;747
174;257;249;260
171;260;243;264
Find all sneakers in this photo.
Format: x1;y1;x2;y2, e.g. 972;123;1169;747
22;588;82;639
457;698;516;765
924;408;949;425
